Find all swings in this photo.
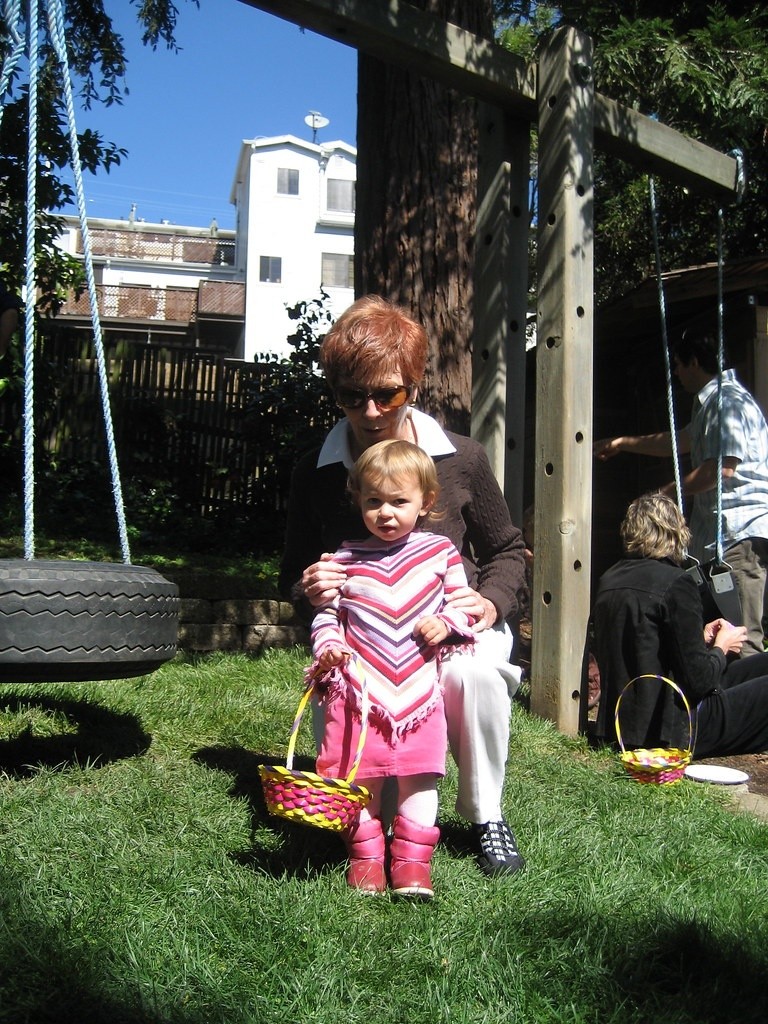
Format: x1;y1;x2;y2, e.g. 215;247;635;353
0;1;182;683
648;164;748;629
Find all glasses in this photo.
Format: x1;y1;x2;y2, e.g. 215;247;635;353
332;382;415;409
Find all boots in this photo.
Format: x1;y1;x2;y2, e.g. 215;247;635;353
390;814;440;898
338;813;386;899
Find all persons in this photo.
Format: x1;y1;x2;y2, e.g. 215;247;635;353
310;439;475;902
279;295;524;877
593;324;768;659
591;494;768;757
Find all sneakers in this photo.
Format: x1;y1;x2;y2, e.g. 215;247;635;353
472;819;526;880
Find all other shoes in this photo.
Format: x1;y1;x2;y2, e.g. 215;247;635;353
587;655;601;707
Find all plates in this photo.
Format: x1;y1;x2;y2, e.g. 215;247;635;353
685;765;750;784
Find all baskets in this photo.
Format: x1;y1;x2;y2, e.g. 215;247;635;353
257;650;373;831
615;675;691;786
